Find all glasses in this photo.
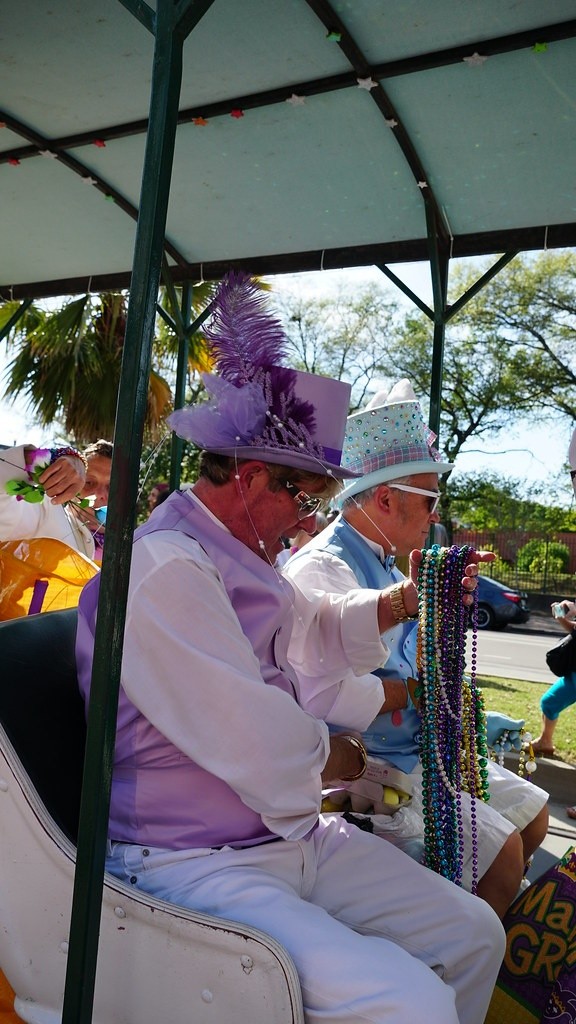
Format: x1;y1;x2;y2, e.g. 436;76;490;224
372;484;442;514
267;466;339;533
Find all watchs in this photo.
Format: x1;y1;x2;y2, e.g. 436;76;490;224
390;580;419;623
340;735;368;781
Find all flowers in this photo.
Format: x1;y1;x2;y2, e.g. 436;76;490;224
4;447;97;509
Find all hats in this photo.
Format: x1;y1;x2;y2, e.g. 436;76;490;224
328;378;455;503
165;269;364;479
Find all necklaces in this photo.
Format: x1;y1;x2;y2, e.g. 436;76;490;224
416;545;490;895
491;726;537;782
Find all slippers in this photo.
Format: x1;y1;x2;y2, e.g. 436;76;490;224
520;743;556;757
568;806;576;819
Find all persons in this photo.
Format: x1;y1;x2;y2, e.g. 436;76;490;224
276;509;339;567
521;602;576;817
277;380;550;918
0;442;195;622
75;283;506;1023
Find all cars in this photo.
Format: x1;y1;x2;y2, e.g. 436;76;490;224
469;575;531;630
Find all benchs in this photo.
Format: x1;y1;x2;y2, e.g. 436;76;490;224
0;606;305;1024
